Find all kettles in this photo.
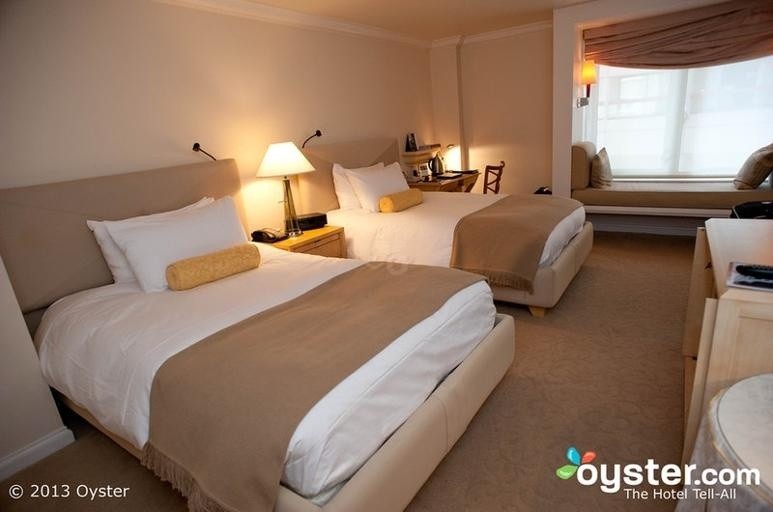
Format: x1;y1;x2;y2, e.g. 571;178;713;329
428;153;445;175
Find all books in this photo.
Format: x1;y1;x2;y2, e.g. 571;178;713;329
727;262;773;291
453;170;478;174
438;173;463;178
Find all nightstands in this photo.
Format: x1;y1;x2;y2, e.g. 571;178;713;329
268;225;347;259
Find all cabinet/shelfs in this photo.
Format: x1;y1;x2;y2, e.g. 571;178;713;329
681;217;773;484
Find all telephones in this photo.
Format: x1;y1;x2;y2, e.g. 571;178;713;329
251;227;288;242
405;175;421;183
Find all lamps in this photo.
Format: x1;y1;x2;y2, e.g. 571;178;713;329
257;141;317;236
577;59;597;108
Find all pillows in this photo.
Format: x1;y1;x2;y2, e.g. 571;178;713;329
332;160;424;214
83;197;261;290
733;144;773;190
591;147;612;188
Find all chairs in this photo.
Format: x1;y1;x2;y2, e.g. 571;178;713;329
483;160;506;193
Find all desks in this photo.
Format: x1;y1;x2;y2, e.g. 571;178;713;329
405;170;483;192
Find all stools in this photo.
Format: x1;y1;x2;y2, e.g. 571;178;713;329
673;370;772;512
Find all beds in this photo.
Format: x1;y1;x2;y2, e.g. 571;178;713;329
0;160;516;512
571;140;773;236
283;135;593;320
